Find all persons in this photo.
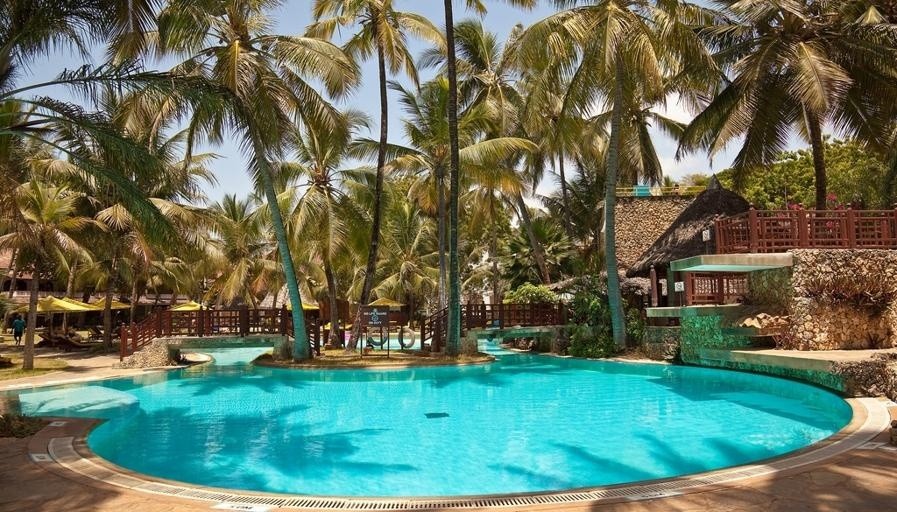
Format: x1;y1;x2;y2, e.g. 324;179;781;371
12;315;26;345
68;330;81;342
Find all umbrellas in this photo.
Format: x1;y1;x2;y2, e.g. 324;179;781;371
95;296;131;310
61;296;104;311
10;295;102;336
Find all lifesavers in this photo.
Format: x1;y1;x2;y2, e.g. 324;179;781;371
397;326;416;349
368;326;388;345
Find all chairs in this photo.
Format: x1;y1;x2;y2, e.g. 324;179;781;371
38;325;118;348
